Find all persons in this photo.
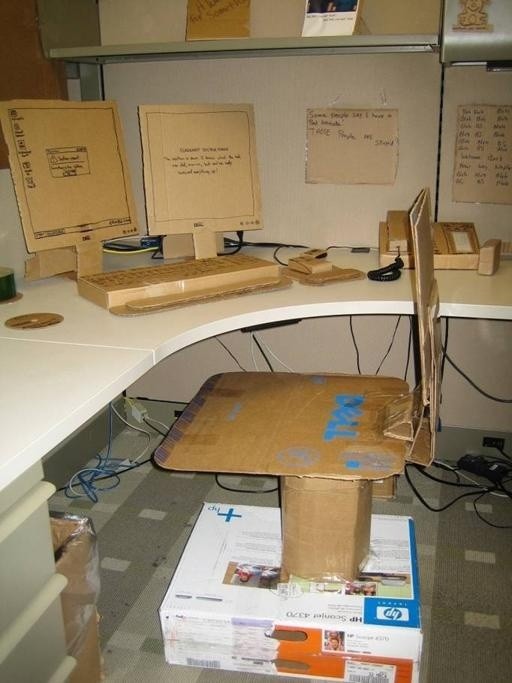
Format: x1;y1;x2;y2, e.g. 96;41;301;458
325;639;344;651
235;563;279;588
328;632;342;650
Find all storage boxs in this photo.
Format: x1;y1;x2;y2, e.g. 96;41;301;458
48;508;99;651
66;610;102;682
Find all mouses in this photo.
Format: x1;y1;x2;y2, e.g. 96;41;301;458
289;257;330;276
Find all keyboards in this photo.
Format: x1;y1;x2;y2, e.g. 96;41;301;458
78;257;277;308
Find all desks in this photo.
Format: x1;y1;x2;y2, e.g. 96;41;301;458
0;243;510;682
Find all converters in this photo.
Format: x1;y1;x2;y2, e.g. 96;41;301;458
456;454;508;484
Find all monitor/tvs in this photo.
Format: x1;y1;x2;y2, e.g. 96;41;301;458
0;98;138;277
136;104;262;257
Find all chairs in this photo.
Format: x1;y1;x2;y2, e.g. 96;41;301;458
149;185;444;682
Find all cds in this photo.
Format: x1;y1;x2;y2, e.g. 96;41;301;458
6;314;63;329
